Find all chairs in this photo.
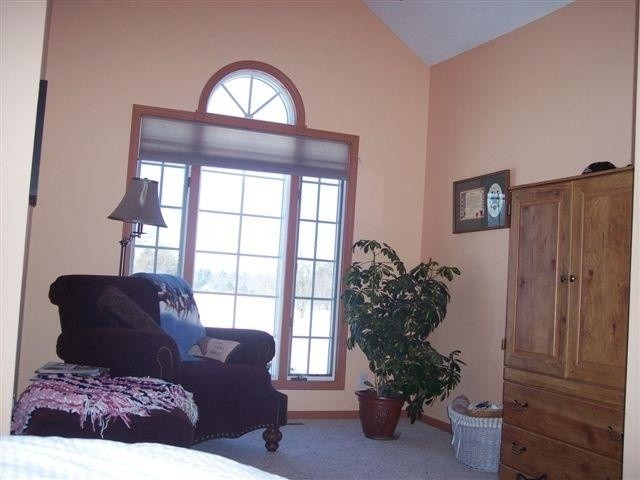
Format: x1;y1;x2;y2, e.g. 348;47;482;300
46;271;288;454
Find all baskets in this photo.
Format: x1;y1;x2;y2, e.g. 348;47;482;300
448;397;502;473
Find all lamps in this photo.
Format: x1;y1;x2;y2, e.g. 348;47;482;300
105;176;170;276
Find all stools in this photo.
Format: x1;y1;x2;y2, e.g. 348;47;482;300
28;374;196;451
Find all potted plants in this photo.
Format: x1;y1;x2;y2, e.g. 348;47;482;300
340;239;470;441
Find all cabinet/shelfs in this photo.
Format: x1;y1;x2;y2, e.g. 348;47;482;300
496;164;633;478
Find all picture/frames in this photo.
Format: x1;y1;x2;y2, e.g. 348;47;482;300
451;171;511;234
